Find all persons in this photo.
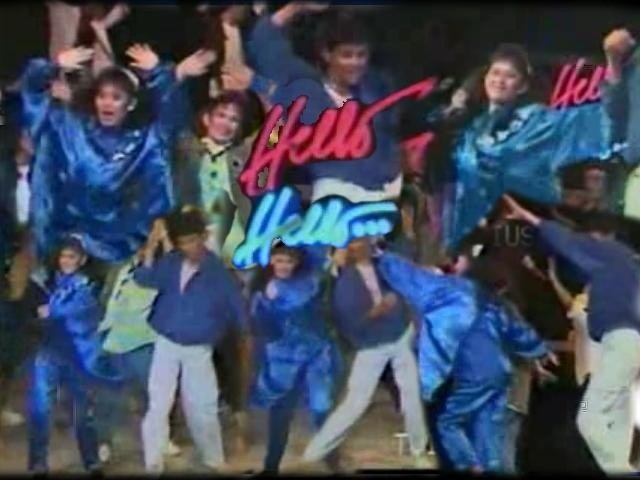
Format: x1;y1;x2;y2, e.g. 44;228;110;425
0;1;640;476
